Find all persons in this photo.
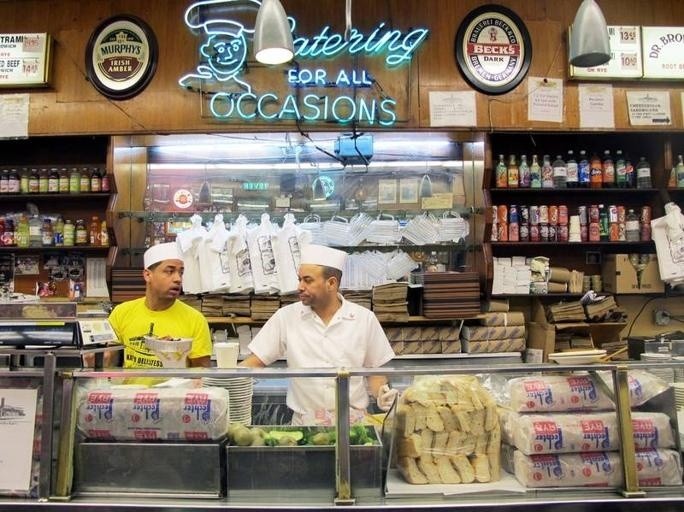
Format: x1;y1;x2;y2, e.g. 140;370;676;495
83;241;213;367
237;243;396;427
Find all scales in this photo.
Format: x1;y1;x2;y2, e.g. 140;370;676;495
0;312;122;350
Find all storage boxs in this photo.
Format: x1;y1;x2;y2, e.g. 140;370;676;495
601;252;668;294
528;319;631;362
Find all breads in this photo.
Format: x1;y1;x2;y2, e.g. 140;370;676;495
395;374;500;485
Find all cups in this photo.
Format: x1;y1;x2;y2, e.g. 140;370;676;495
214;343;240;368
299;211;471;290
583;275;602;293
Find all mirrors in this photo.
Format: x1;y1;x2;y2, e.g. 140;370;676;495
127;132;475;274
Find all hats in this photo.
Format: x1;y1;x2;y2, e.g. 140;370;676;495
297;243;349;274
143;241;184;270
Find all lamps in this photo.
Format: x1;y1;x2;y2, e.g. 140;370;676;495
198;168;212;207
313;163;328;202
569;0;611;67
253;0;294;66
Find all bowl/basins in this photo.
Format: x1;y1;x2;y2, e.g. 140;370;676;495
143;334;192;352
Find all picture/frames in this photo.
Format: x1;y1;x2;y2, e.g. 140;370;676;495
377;178;397;205
399;178;418;204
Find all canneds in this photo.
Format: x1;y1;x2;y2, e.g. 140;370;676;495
491;205;652;242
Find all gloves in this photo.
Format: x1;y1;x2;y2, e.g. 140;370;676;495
377;384;400;413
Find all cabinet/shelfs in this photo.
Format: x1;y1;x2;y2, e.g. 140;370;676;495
0;133;117;253
492;129;682;300
0;356;684;512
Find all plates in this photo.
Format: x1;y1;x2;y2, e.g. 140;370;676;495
313;176;334;196
639;351;684;452
545;349;608;364
199;377;253;426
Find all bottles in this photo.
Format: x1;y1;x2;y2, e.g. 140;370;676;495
0;166;108;246
598;205;609;242
493;151;684;188
68;267;82;303
626;209;640;242
